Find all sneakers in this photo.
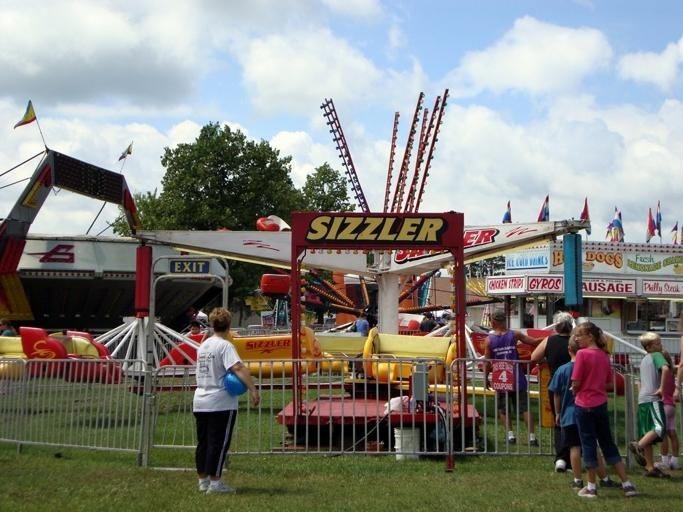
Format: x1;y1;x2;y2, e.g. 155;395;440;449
530;438;539;447
206;483;235;492
629;441;647;466
670;462;681;470
555;460;637;497
645;468;670;480
507;437;516;445
199;481;209;492
654;462;670;470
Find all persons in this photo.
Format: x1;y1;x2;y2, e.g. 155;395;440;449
184;321;208;342
354;313;369;338
420;310;454;334
526;310;683;500
482;310;545;446
191;307;261;496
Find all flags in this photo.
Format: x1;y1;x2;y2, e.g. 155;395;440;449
538;193;549;221
579;197;591;234
503;200;512;223
602;198;683;247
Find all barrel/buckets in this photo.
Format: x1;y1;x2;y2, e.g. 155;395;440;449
393;427;421;461
